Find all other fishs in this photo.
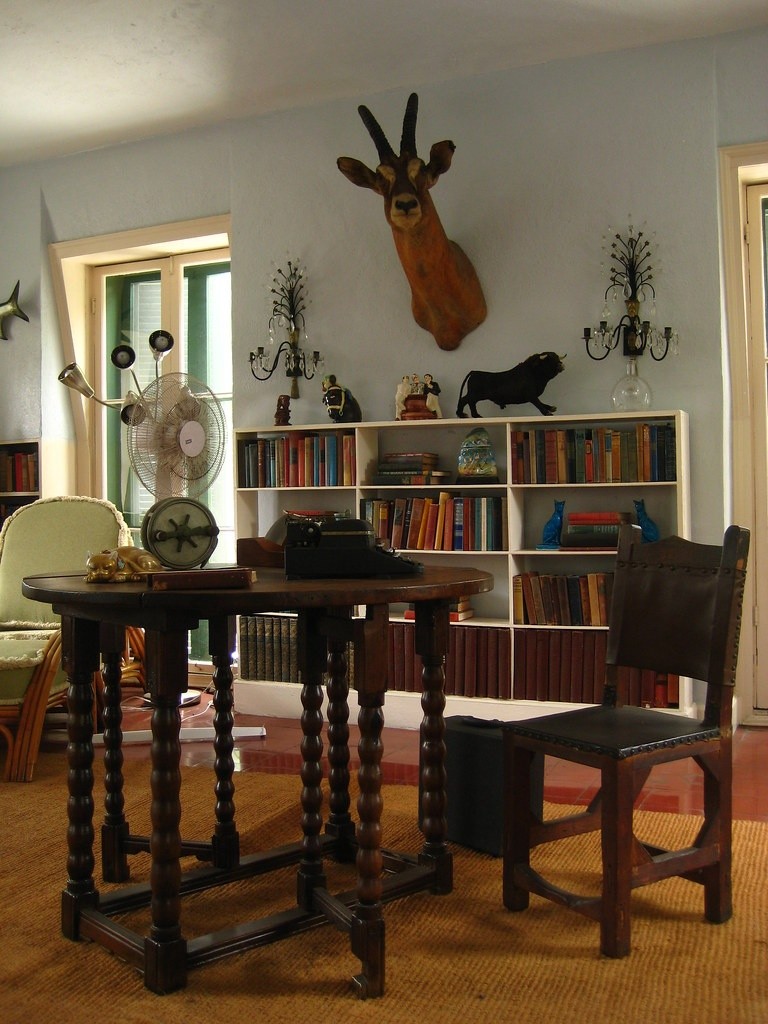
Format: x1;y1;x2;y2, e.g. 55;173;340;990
0;280;29;340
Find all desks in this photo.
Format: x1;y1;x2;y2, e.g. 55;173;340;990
21;561;494;999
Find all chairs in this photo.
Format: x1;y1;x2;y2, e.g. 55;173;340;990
502;511;750;957
0;494;146;782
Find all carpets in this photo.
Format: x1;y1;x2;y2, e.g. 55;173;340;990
0;753;768;1024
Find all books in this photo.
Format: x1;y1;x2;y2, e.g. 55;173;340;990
0;451;38;529
238;422;675;625
236;617;680;711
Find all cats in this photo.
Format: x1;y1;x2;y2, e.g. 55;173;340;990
543;499;566;545
633;499;661;544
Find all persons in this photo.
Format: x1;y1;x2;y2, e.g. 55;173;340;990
410;373;423;394
394;375;412;420
421;374;443;418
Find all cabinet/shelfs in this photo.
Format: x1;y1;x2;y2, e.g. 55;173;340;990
233;407;695;731
0;439;39;532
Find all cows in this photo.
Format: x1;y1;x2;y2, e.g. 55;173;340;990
456;352;567;419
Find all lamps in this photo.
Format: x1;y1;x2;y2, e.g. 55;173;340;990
111;345;153;419
580;213;679;375
57;362;120;411
148;330;174;419
247;262;324;399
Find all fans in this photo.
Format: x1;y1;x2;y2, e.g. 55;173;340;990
127;372;227;500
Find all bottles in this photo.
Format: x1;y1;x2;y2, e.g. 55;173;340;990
613;359;651;412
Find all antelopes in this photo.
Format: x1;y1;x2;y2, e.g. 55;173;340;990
337;93;487;352
